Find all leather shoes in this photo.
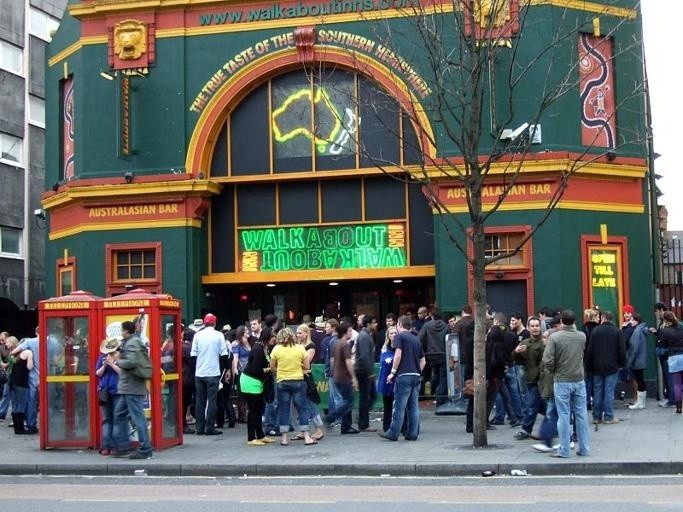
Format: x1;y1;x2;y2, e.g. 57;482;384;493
184;416;235;435
341;427;397;441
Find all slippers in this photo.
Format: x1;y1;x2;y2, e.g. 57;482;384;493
280;432;324;445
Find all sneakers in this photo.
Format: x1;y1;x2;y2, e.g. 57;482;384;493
532;434;580;458
101;450;110;455
260;437;274;443
657;398;676;408
29;429;38;435
128;450;153;460
488;418;529;439
247;439;266;445
592;420;602;424
604;419;620;424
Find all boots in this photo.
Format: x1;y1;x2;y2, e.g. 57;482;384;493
676;400;682;413
14;412;29;434
629;380;648;409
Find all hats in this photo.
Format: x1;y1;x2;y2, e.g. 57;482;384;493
188;318;204;332
622;305;635;314
204;313;216;325
99;337;122;354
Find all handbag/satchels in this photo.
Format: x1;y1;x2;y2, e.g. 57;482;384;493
463;377;474;395
264;372;275;403
98;387;109;406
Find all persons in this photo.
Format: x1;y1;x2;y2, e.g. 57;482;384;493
163;312;377;445
378;305;474;441
542;308;590;458
0;324;89;434
621;304;682;414
94;335;120;456
484;305;627;450
112;320;152;459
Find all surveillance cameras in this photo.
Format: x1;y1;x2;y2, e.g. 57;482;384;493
506;123;529;142
